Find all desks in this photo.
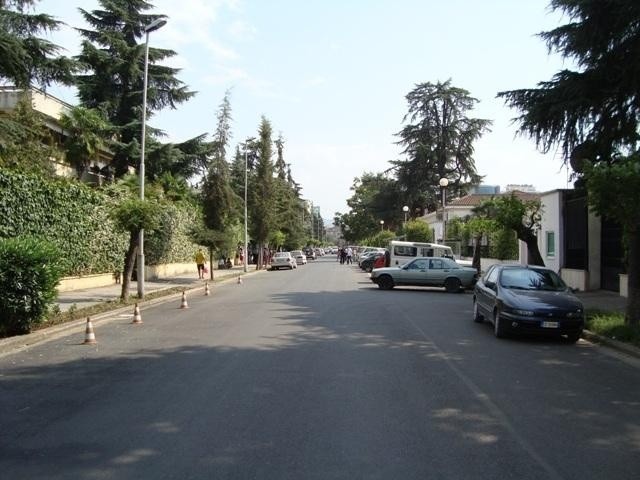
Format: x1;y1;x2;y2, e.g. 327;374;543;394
402;205;409;243
136;15;167;300
379;219;384;236
244;136;257;273
439;177;449;246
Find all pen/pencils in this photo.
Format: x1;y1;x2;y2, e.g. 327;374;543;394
383;240;455;266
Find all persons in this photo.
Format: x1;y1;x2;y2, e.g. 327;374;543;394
239;248;244;265
194;248;205;280
227;258;232;268
336;247;353;265
218;256;224;266
263;248;269;268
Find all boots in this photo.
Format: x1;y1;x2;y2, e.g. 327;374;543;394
301;246;316;260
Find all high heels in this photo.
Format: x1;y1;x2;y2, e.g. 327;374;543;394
177;290;191;309
82;315;100;346
130;302;144;325
237;275;243;285
203;282;212;296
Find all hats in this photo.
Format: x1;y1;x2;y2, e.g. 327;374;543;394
314;245;385;273
290;250;307;265
472;262;584;343
369;256;479;293
270;252;297;270
